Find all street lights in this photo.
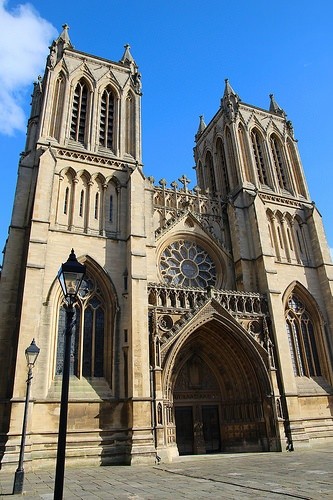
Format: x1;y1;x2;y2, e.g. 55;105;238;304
10;338;40;495
51;248;89;500
